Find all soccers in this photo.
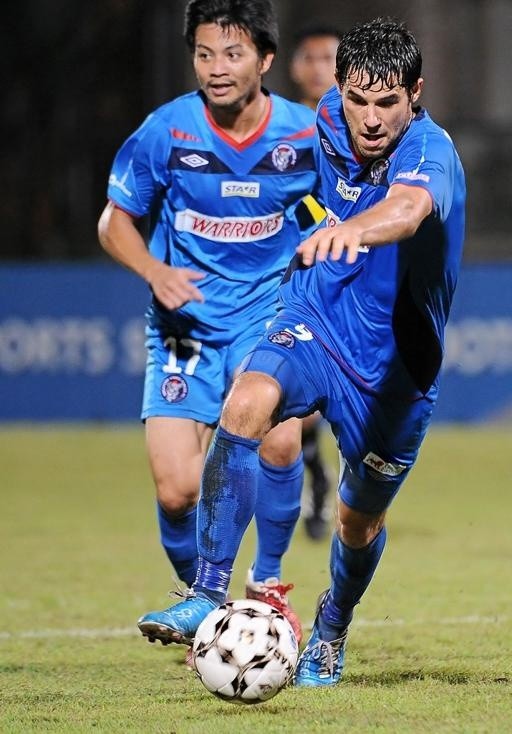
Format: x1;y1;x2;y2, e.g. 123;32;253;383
193;600;299;705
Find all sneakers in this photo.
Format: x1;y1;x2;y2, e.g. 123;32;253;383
244;563;303;644
304;478;332;541
295;587;353;685
138;592;217;647
184;595;234;673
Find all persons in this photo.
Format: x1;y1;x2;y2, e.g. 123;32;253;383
97;0;322;649
296;23;341;544
131;18;468;693
